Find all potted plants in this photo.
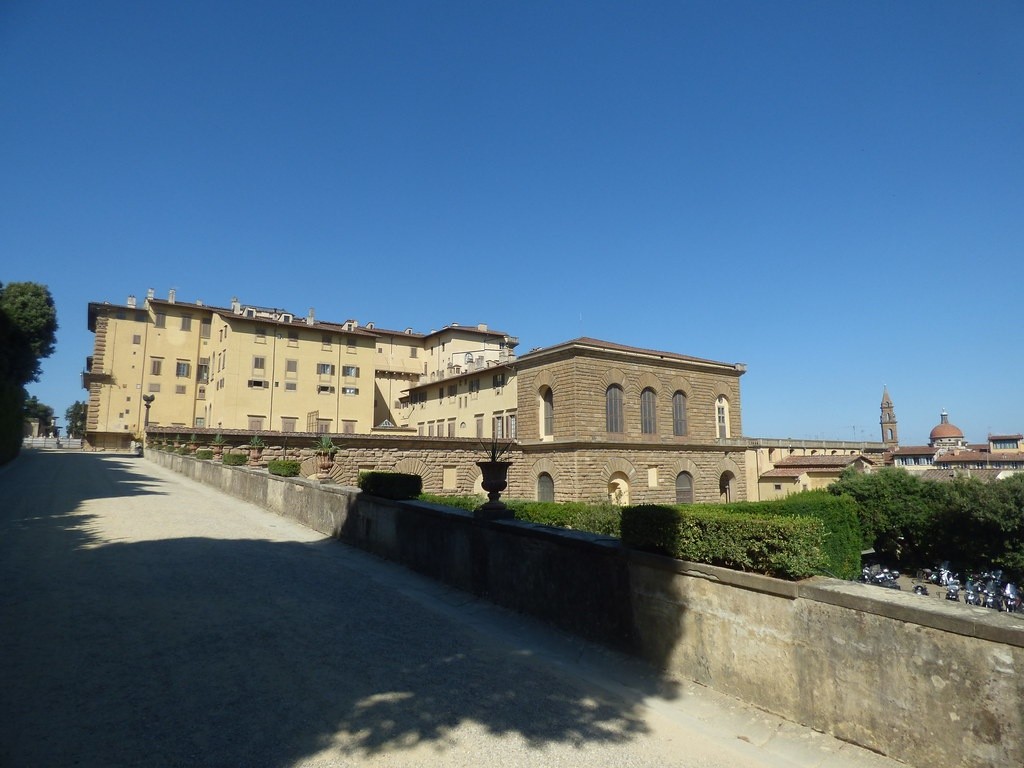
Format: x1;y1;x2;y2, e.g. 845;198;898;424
154;436;160;443
188;434;202;451
248;434;265;462
161;436;168;445
310;435;336;470
474;437;516;491
172;435;182;448
210;432;230;457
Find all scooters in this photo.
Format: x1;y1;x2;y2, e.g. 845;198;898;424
859;565;1021;613
